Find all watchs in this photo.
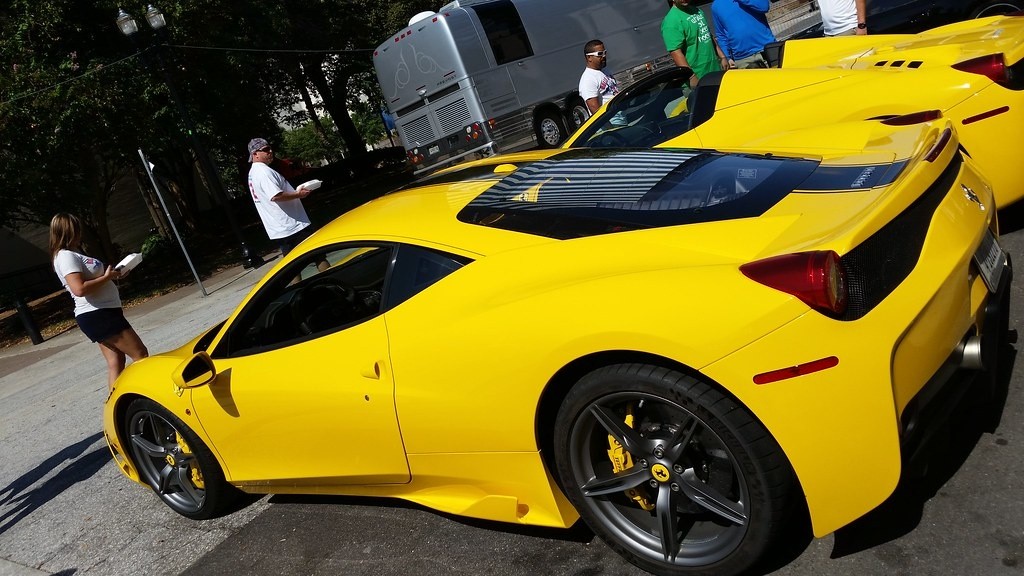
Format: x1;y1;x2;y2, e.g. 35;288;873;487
857;23;868;29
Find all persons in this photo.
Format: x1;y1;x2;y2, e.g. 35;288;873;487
817;0;868;38
660;0;727;97
246;137;331;285
579;40;628;133
711;0;774;69
49;211;148;393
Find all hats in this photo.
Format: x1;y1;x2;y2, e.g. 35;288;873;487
246;136;271;161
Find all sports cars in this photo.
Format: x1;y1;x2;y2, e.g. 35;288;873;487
560;13;1024;210
80;113;1005;576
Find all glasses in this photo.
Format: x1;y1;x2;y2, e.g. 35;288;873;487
260;148;271;151
585;49;607;57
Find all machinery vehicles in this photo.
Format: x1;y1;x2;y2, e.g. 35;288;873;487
372;0;720;176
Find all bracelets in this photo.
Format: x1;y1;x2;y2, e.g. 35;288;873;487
719;56;725;60
690;73;695;80
